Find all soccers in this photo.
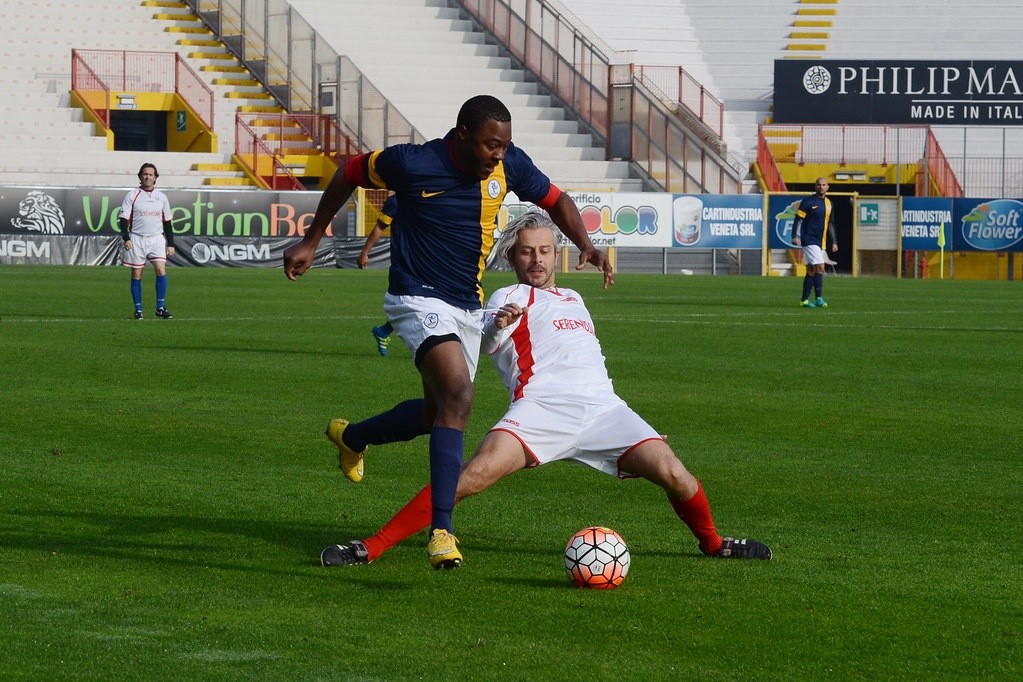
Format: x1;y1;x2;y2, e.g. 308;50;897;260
564;526;631;589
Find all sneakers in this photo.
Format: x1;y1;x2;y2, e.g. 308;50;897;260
699;536;772;560
815;297;827;307
801;301;815;308
325;419;368;484
320;539;373;570
155;309;171;318
134;311;142;319
427;528;463;570
372;326;390;356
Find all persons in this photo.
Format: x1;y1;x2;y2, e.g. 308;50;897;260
321;211;773;568
118;163;175;319
284;95;614;569
792;177;838;308
